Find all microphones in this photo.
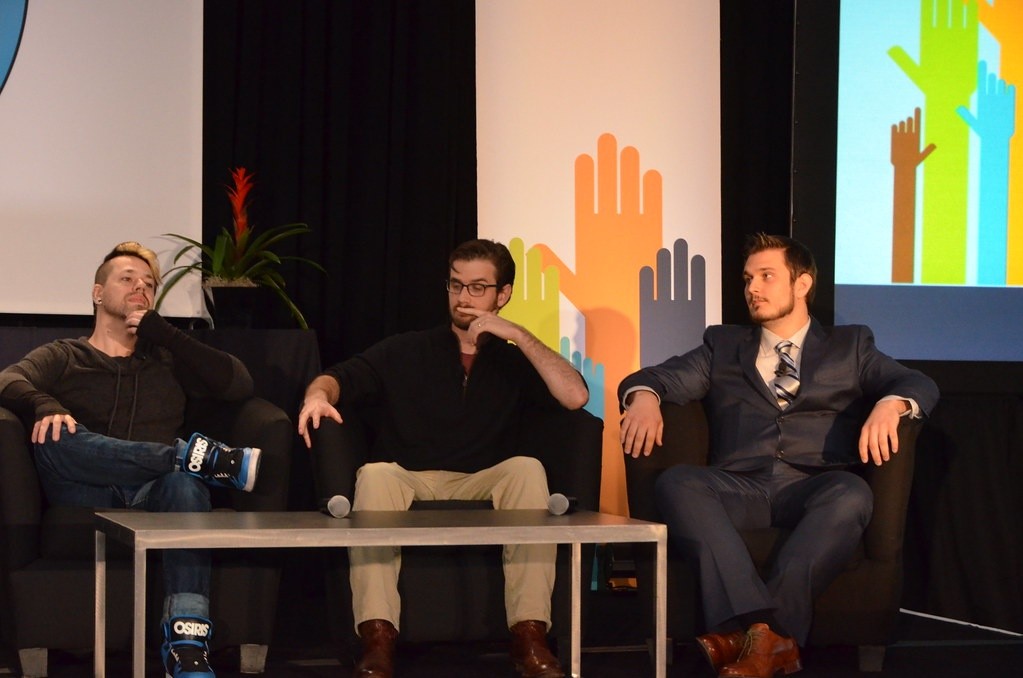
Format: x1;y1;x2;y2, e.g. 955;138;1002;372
321;494;350;517
547;493;578;515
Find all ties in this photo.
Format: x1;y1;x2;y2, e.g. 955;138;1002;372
773;341;801;412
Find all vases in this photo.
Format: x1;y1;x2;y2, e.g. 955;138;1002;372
205;282;276;338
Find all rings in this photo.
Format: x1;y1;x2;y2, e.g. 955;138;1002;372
478;322;480;327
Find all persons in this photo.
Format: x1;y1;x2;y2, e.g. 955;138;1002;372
0;241;263;678
298;240;590;678
617;234;940;678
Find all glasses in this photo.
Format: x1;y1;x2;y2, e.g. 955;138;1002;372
446;280;499;297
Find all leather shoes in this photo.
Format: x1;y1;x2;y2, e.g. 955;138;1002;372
720;621;803;678
694;628;746;673
351;619;398;678
509;620;565;678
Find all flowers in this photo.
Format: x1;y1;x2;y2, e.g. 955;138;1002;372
152;167;323;331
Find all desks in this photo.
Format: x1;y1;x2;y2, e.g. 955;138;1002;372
94;512;668;678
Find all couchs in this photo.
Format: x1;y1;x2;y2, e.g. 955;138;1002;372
618;396;927;672
0;398;295;676
306;386;604;667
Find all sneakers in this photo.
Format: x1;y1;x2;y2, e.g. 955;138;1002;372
183;432;263;493
159;613;216;678
